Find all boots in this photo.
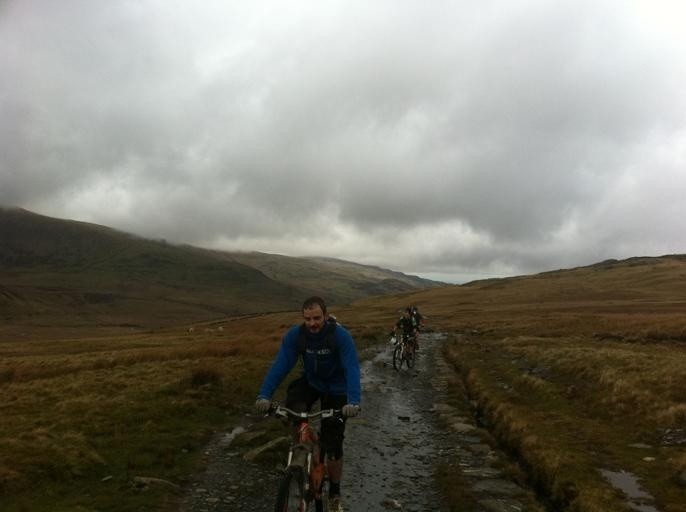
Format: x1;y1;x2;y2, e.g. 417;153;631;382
328;480;349;512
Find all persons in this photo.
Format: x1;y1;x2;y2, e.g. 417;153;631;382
408;307;425;351
389;308;417;360
254;296;362;511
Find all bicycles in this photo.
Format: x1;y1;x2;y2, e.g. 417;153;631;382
263;401;361;512
390;333;417;372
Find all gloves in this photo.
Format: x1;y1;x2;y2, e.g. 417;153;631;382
255;398;271;413
341;404;359;417
